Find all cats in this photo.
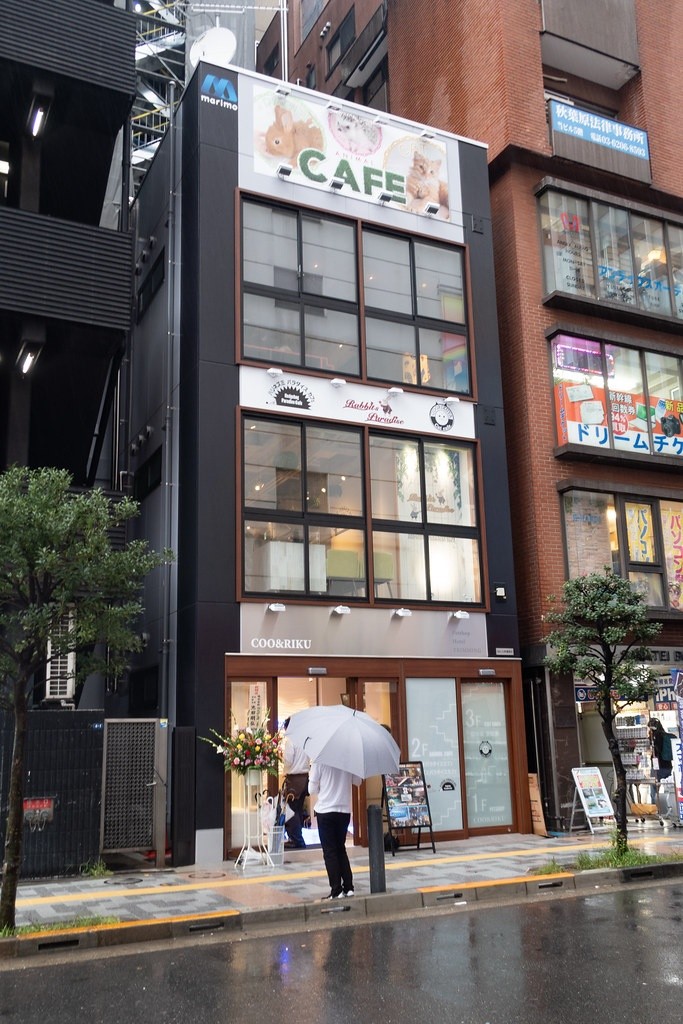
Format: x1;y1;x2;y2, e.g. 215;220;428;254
406;151;449;209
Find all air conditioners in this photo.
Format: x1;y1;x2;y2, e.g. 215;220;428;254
44;602;76;701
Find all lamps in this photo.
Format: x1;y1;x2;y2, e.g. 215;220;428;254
419;130;437;140
373;116;390;127
275;85;291;98
14;322;48;380
268;603;287;613
307;667;328;676
478;669;496;676
453;610;470;620
266;368;284;378
333;605;351;616
396;607;412;618
424;202;440;218
377;192;392;207
329;179;344;195
277;165;292;182
387;387;403;395
443;396;460;404
330;377;347;388
319;21;331;38
23;76;56;142
325;100;343;113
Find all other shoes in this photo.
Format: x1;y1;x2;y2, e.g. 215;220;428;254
345;890;355;897
321;890;344;900
284;841;298;849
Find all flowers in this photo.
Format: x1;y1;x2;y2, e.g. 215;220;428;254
194;706;288;778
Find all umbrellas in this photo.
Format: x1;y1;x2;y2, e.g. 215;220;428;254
287;705;401;781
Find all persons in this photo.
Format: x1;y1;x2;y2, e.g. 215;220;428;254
380;724;401;764
647;718;673;820
308;761;362;902
282;716;308;850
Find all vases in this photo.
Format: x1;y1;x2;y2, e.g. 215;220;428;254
243;766;262;787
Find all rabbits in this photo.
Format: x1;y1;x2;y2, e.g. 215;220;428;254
262;105;326;172
